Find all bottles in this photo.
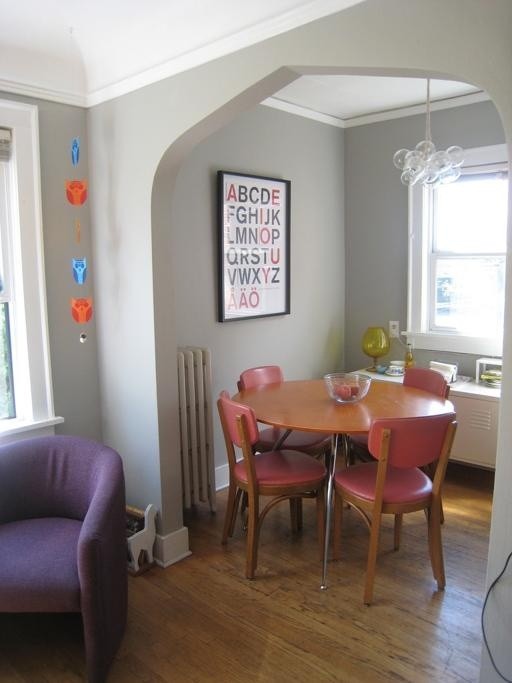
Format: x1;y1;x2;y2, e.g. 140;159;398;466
404;343;415;375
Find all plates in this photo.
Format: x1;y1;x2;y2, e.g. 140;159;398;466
385;371;406;376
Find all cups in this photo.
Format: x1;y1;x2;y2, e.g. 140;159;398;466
389;366;404;373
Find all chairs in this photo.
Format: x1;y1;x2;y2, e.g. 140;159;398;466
1;434;130;681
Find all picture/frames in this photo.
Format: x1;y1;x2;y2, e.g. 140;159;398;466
216;169;291;323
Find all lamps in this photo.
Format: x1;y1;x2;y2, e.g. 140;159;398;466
393;76;465;190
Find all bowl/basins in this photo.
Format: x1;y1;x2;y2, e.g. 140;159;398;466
323;373;372;404
375;365;387;374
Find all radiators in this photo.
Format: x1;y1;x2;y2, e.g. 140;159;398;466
176;344;218;513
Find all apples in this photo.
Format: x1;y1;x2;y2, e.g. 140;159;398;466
332;380;361;401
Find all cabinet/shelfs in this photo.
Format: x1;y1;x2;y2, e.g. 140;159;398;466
344;364;496;472
